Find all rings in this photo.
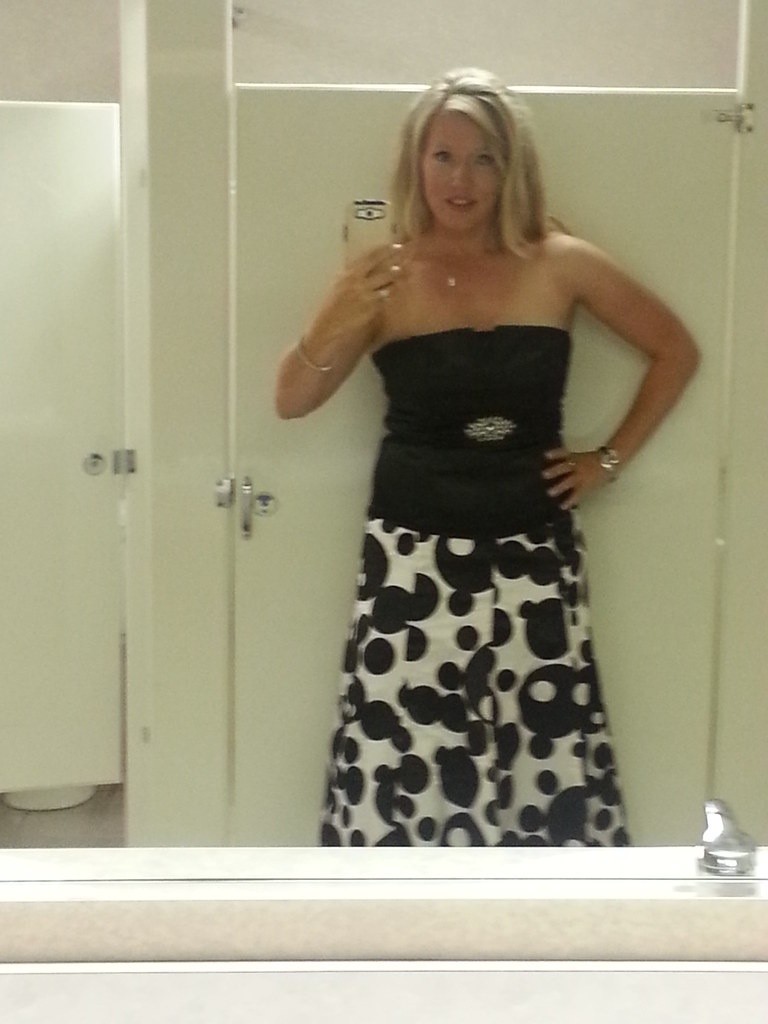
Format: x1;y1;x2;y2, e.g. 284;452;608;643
380;287;391;297
562;459;577;471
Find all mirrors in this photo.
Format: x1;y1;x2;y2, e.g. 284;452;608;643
1;1;766;848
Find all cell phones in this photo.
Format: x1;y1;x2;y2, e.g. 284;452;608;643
345;199;390;258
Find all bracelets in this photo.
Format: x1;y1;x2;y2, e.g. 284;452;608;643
295;335;338;371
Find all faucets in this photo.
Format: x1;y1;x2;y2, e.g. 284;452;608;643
691;799;759;898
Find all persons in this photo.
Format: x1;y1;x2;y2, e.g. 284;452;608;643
276;68;700;847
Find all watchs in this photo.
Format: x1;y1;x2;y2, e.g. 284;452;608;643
600;446;619;481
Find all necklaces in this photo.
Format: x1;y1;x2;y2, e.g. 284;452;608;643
441;265;456;286
